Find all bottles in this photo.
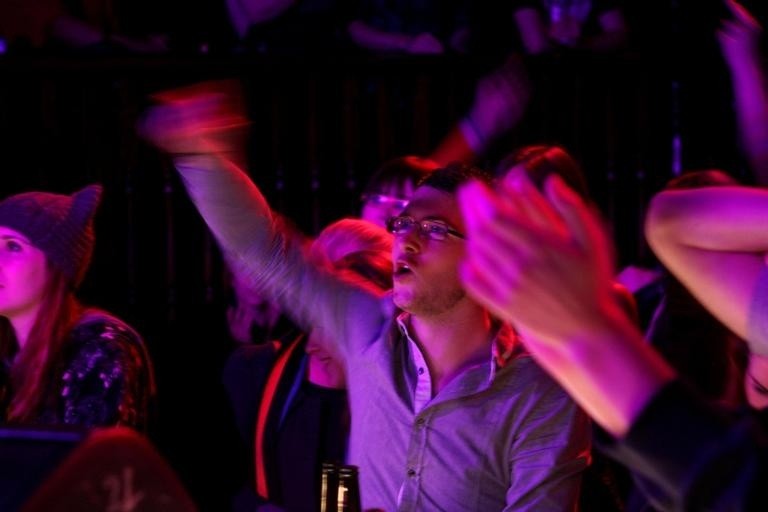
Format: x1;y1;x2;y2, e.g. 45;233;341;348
315;463;361;512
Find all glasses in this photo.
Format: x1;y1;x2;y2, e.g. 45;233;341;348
387;215;465;241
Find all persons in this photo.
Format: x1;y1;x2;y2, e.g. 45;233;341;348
1;0;766;510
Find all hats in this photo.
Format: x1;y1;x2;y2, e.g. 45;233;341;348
1;184;100;287
